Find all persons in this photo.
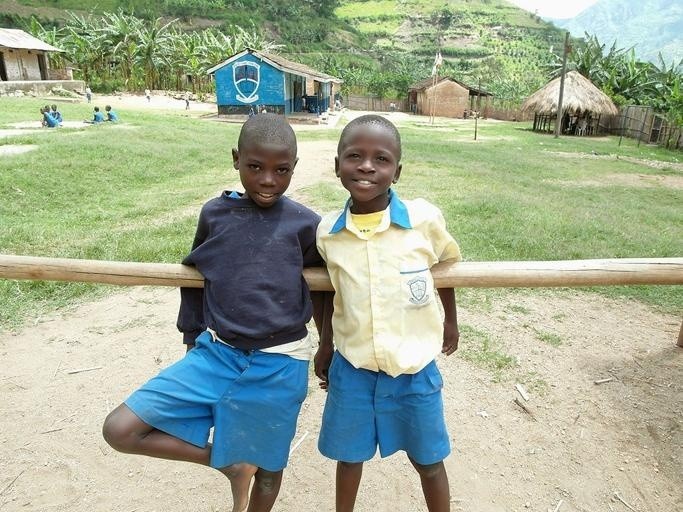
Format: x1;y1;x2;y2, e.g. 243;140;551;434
91;105;118;122
185;100;190;110
247;104;267;117
86;84;92;104
144;86;151;102
313;114;459;511
102;114;329;512
40;104;62;128
